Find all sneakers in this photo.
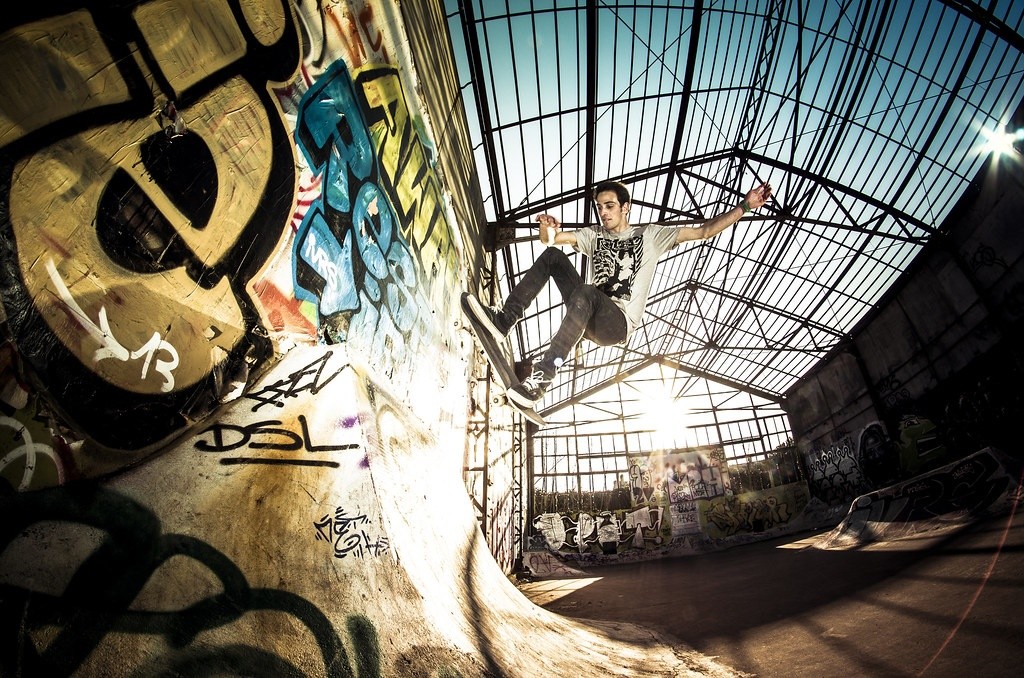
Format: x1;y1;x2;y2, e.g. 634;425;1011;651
506;366;555;409
461;290;516;343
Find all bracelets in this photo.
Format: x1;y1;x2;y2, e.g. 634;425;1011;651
740;200;752;212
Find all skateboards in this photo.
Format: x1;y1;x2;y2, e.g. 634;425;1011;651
460;291;548;427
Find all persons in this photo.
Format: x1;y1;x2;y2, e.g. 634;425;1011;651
463;183;772;409
